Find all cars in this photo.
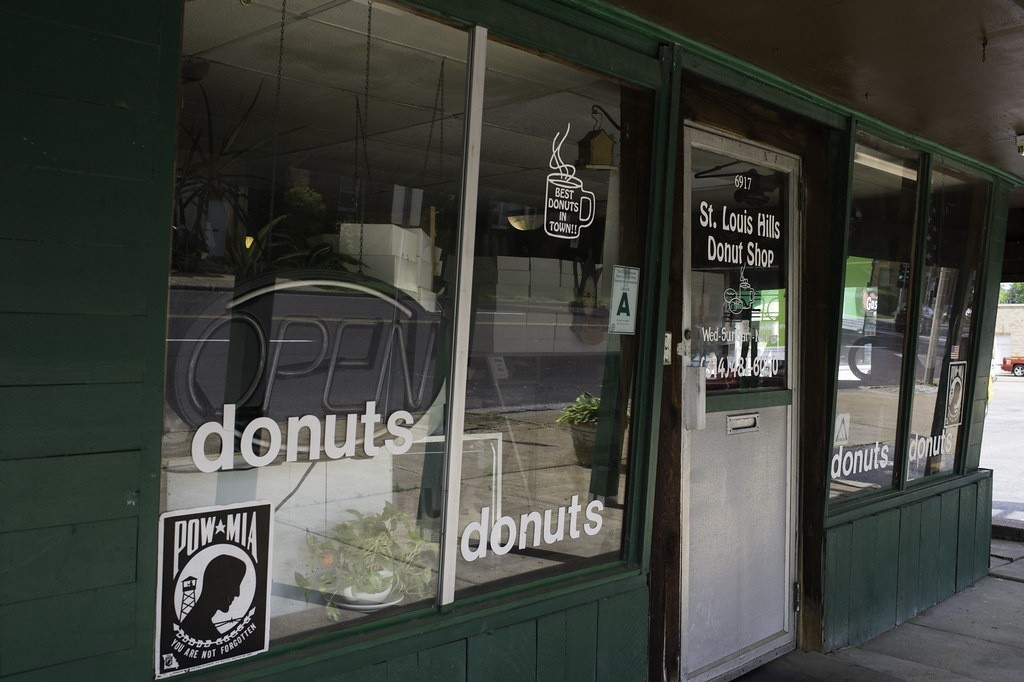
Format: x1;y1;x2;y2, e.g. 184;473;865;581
1001;356;1024;376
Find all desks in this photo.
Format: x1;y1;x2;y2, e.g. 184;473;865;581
160;431;505;621
158;267;443;426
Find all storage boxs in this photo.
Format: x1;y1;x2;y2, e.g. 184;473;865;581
339;223;727;377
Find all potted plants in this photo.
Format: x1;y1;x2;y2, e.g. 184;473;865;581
555;390;630;470
174;68;362;283
293;501;439;624
